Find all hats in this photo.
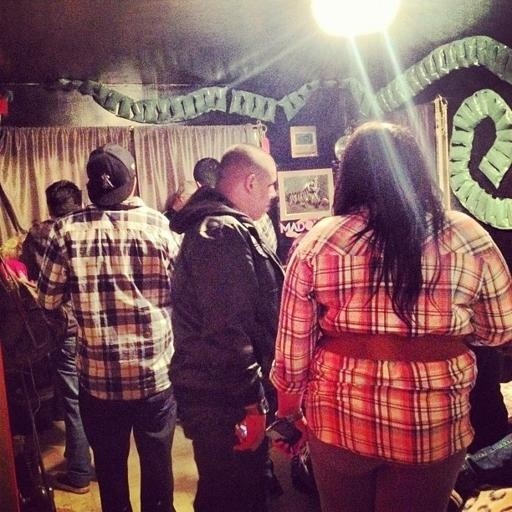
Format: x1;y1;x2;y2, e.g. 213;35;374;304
86;146;136;207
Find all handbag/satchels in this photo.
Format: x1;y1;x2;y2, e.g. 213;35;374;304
10;278;60;365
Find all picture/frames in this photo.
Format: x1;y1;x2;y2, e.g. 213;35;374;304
275;127;335;223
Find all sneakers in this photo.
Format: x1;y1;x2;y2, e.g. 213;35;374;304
450;465;473;508
55;474;90;494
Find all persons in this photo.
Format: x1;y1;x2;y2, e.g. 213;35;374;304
36;144;179;511
167;157;278;253
269;123;511;512
170;143;286;511
1;180;97;510
